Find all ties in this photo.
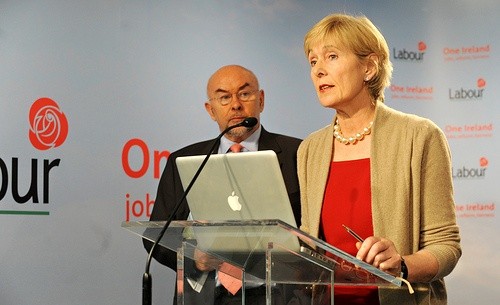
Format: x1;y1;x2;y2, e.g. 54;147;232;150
230;144;244;153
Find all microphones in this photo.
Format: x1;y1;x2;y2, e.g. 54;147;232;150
142;116;258;305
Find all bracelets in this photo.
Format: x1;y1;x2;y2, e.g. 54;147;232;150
401;256;408;286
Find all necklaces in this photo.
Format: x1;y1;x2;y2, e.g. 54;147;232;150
333;119;374;145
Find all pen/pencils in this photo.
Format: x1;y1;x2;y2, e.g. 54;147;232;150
342;224;364;243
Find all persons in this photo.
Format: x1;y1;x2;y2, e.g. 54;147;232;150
142;65;304;305
296;14;462;305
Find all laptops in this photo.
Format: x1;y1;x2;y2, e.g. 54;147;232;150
176;149;377;285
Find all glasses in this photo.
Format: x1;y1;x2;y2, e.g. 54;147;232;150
209;89;259;106
